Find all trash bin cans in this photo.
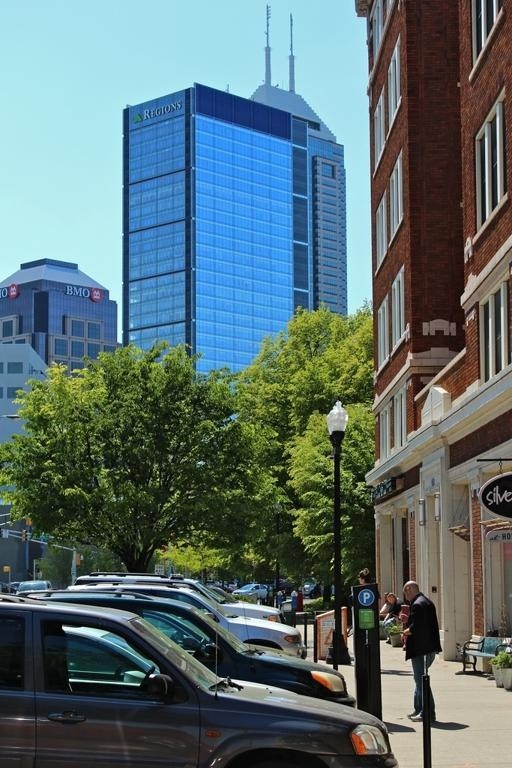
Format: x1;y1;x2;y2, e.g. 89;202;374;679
283;612;295;626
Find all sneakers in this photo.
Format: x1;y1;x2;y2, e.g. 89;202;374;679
407;710;423;722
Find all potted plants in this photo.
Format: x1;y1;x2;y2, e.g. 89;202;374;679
486;650;512;691
386;622;404;649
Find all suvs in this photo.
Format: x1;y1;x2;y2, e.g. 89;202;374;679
62;570;308;660
9;589;357;710
0;592;399;768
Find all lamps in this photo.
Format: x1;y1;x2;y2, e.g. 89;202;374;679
417;491;441;528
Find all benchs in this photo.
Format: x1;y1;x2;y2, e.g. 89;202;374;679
454;636;512;682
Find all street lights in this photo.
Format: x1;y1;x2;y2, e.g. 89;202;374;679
326;400;354;667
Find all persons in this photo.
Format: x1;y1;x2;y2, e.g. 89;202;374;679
378;591;402;614
398;580;442;723
382;592;405;647
357;568;370;584
